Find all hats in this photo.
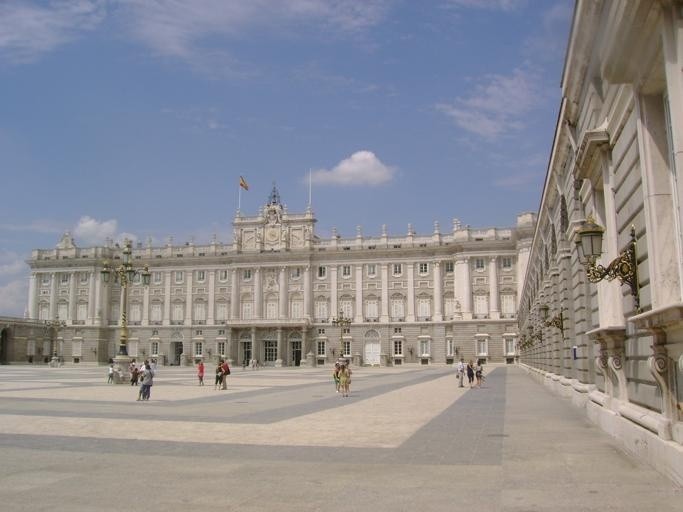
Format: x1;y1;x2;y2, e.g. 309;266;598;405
145;365;151;369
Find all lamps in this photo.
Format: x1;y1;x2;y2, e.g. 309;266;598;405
536;302;564;334
572;212;643;315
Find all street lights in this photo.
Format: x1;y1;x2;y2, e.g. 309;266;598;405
332;308;351;358
46;314;66;356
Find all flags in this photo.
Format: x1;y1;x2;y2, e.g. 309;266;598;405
239;175;250;190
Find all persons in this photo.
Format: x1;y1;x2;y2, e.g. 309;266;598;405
108;359;158;402
475;361;483;389
220;359;230;390
241;357;246;371
333;362;352;399
467;359;475;389
456;358;466;388
214;363;225;391
198;362;206;386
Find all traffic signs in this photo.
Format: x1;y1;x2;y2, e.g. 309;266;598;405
101;240;150;359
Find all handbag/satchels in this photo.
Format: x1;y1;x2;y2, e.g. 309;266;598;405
346;378;351;384
140;376;144;381
